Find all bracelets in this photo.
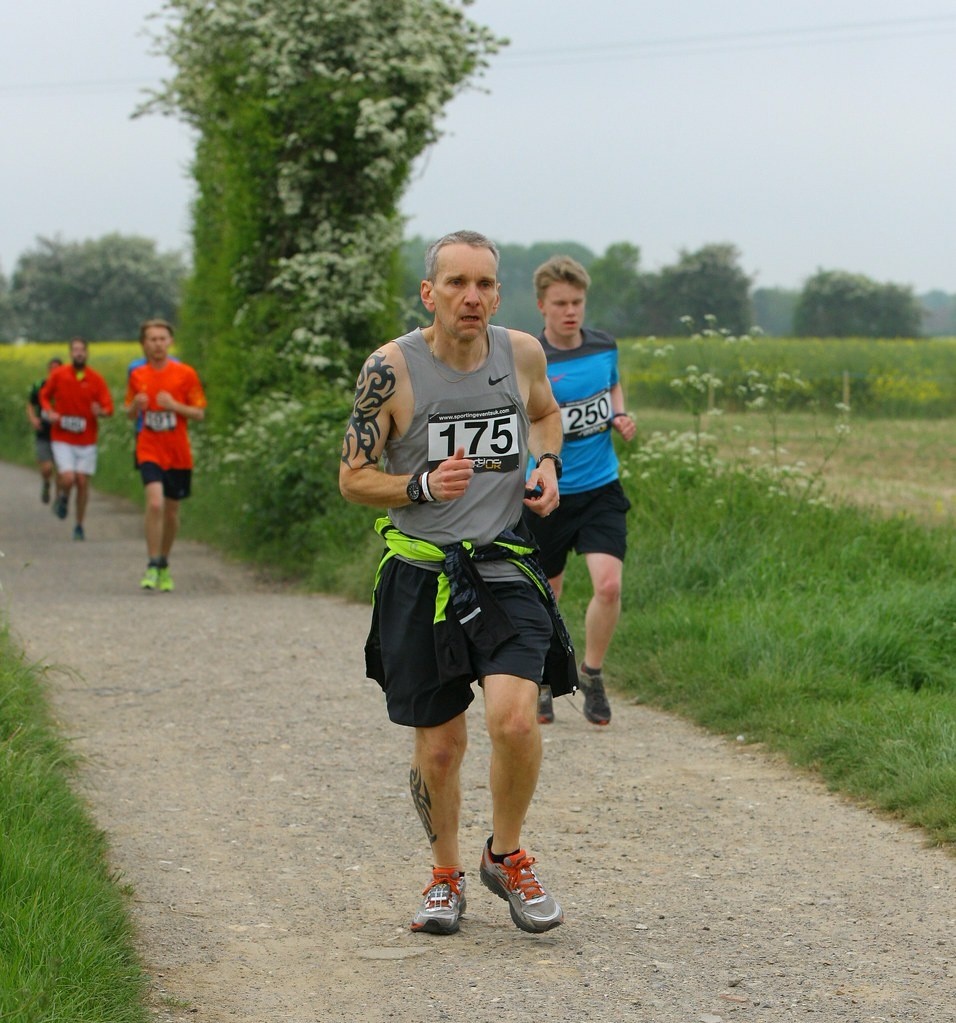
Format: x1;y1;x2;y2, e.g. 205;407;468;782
614;413;628;418
421;470;435;502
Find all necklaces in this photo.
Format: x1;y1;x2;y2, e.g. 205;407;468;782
427;329;483;383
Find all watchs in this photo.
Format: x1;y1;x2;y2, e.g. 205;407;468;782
536;453;563;480
406;472;428;505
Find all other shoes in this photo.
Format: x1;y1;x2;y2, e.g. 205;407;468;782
74;525;84;541
53;498;59;512
40;482;50;503
57;495;68;518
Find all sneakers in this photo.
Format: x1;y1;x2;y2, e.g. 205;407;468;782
578;671;612;725
410;866;467;934
538;685;554;723
479;835;564;934
140;565;158;589
158;565;174;592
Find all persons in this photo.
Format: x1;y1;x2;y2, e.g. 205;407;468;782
336;230;565;932
523;254;637;724
123;319;207;591
26;338;115;543
